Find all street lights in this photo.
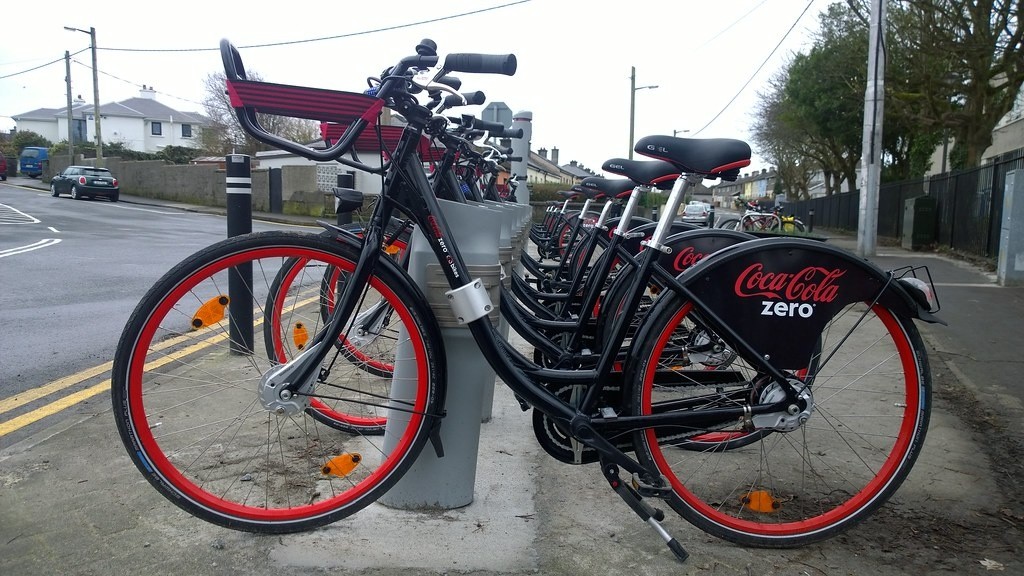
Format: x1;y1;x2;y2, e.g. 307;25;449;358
64;25;104;168
628;84;659;160
673;129;691;140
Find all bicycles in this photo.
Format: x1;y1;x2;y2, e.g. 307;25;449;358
110;36;951;565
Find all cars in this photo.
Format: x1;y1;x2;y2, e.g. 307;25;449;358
689;200;711;218
0;151;8;181
49;166;119;201
680;204;709;226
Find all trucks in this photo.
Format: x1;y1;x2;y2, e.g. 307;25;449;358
18;147;52;177
